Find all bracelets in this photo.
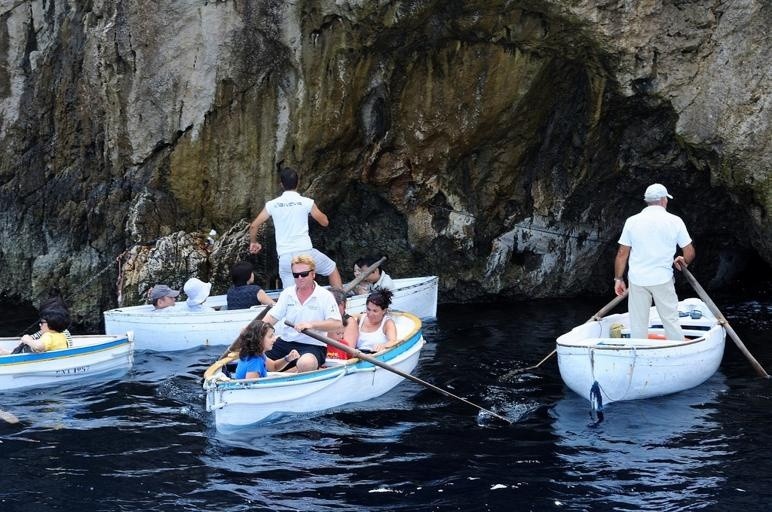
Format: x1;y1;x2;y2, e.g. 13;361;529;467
613;276;623;282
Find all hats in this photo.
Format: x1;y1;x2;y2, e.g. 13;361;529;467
645;182;673;201
150;284;179;300
184;278;212;305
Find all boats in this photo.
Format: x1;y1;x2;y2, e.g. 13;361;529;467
102;276;439;354
0;331;136;391
553;297;726;424
202;308;425;424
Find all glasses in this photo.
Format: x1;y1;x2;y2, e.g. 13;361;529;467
293;269;314;279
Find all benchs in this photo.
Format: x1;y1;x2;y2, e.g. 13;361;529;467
581;330;689;355
618;321;709;337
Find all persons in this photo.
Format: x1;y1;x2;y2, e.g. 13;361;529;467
614;182;696;342
246;166;344;300
226;255;395;381
184;276;218;313
21;307;71;353
26;295;72;349
150;286;182;313
